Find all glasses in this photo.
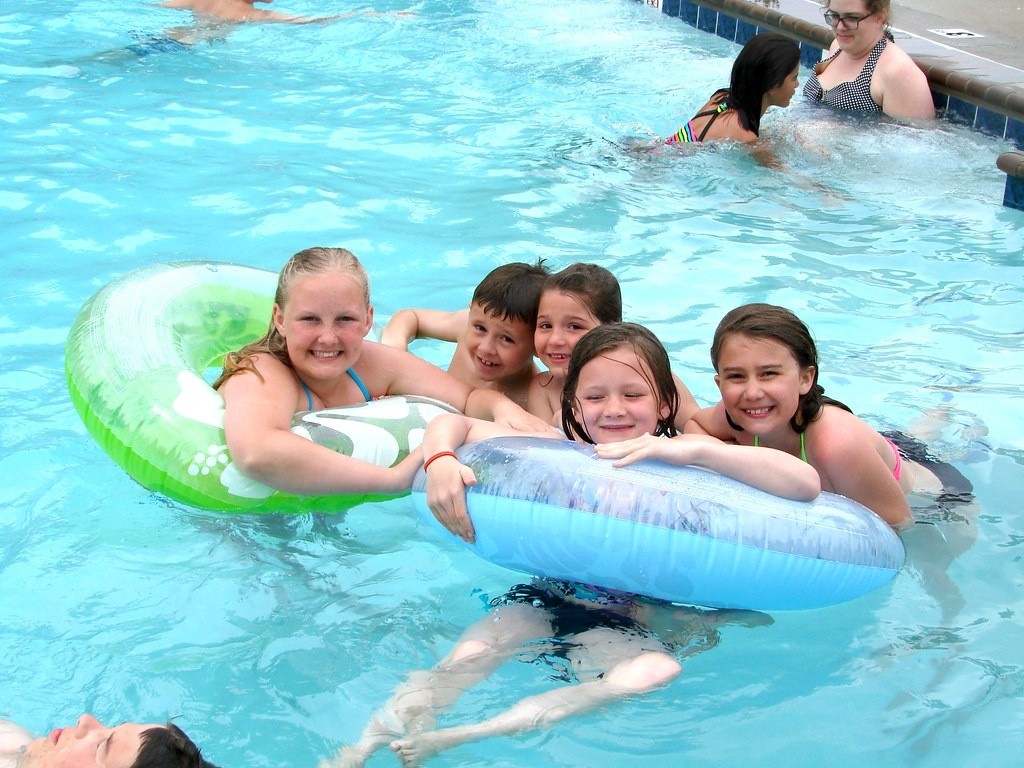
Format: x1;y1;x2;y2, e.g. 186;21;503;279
823;7;879;32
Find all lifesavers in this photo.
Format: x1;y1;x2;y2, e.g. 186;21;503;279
64;259;464;516
412;436;905;610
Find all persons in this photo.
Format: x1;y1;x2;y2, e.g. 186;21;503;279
209;246;570;497
0;710;221;768
128;0;325;64
647;31;827;171
381;254;557;414
679;303;982;566
524;263;703;445
320;321;824;768
803;0;937;125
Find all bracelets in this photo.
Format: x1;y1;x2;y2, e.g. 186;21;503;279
423;449;458;473
